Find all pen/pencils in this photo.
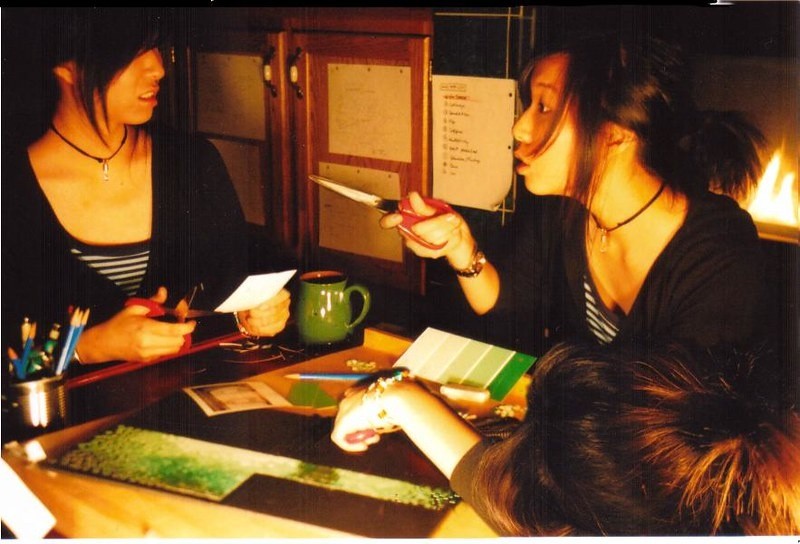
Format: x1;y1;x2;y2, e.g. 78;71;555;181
282;373;371;380
7;304;90;382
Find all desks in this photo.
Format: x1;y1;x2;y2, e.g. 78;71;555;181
1;318;533;539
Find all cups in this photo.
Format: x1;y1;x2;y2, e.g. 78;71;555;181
10;371;72;435
294;269;370;349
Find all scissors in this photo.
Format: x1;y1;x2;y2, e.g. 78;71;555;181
306;175;453;251
123;282;224;358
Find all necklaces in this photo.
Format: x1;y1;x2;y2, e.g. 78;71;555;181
48;120;129;182
586;178;669;253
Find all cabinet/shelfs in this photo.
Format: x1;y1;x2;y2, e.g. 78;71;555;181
175;26;432;296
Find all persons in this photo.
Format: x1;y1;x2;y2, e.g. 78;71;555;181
329;0;800;367
335;337;800;536
1;0;291;388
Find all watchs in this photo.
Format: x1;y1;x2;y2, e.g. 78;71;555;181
451;244;485;279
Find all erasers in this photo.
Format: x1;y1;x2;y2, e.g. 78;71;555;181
440;384;492;403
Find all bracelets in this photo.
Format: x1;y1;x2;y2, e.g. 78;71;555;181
70;350;83;369
233;310;249;336
362;367;426;433
